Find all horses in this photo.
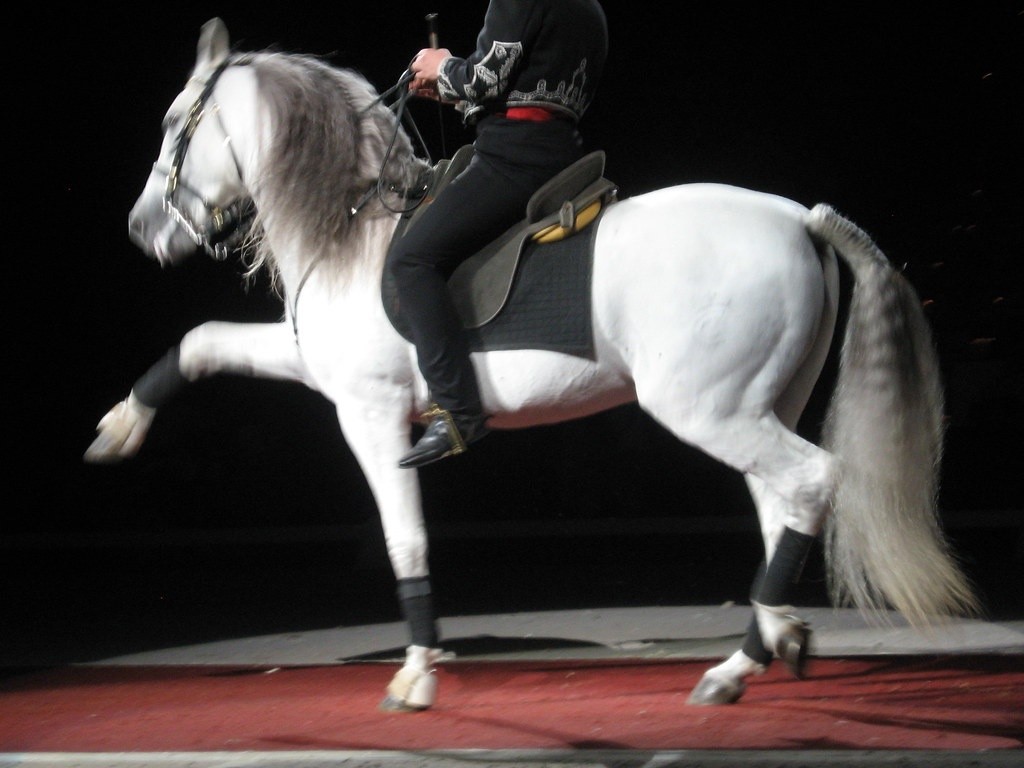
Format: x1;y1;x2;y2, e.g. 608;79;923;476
79;16;988;707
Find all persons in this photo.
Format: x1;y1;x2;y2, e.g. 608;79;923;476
390;0;608;467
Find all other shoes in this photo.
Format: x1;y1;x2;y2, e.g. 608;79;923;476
394;413;491;470
